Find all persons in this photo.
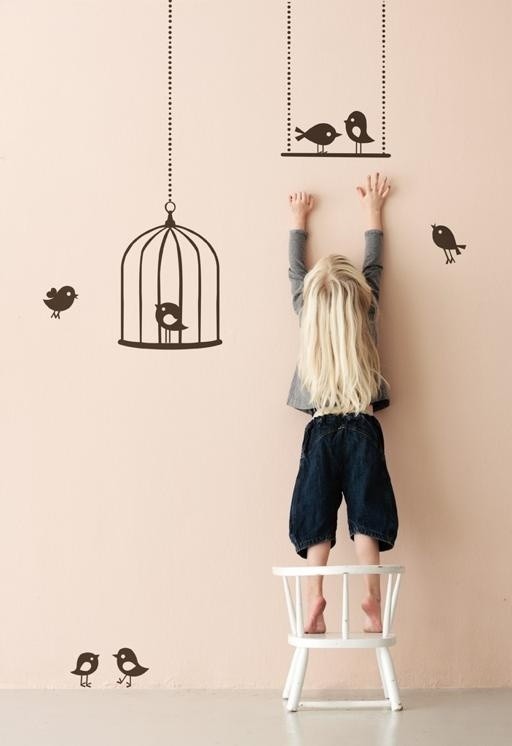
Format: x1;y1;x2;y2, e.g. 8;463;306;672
289;171;391;634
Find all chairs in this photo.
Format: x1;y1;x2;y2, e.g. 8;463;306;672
270;563;407;712
